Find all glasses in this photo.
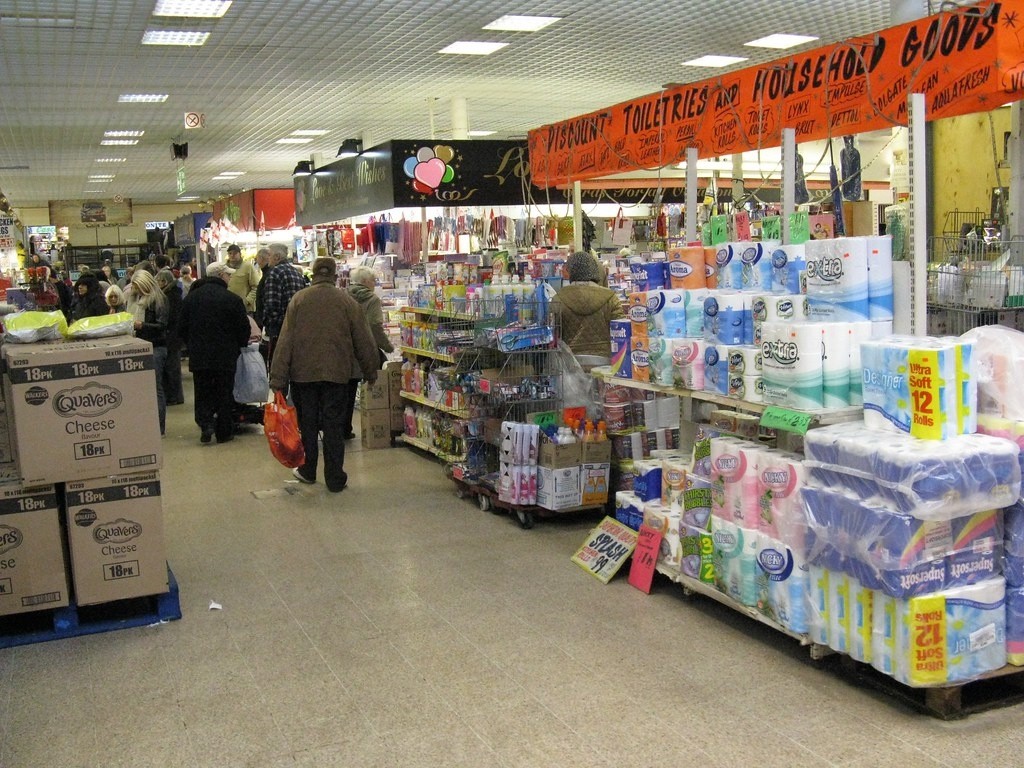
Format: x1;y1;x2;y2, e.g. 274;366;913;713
109;295;118;298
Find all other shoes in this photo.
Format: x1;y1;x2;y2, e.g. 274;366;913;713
293;467;315;484
346;433;355;440
215;434;234;443
166;401;183;406
201;428;215;442
344;474;348;487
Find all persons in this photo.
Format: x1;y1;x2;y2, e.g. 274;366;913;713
548;252;626;375
0;244;393;492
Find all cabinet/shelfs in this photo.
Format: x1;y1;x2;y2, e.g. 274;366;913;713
926;208;1024;337
399;281;562;507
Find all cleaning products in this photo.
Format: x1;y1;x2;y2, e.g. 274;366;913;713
936;260;960;307
401;272;611;508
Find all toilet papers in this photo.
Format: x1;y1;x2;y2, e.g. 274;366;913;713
591;234;1023;663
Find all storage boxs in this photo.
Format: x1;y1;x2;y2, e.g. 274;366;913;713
949;249;1024;308
0;334;170;617
525;410;614;509
361;360;404;449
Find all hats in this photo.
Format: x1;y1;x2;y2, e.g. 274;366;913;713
567;251;600;284
312;258;336;277
227;244;240;252
207;261;236;277
49;269;57;279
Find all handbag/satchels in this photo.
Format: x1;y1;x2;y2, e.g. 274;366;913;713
609;203;685;247
699;204;834;246
263;390;305;469
295;211;424;267
542;340;601;425
378;348;388;370
234;343;269;403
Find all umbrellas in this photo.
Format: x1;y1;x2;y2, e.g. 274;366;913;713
360;213;388;255
